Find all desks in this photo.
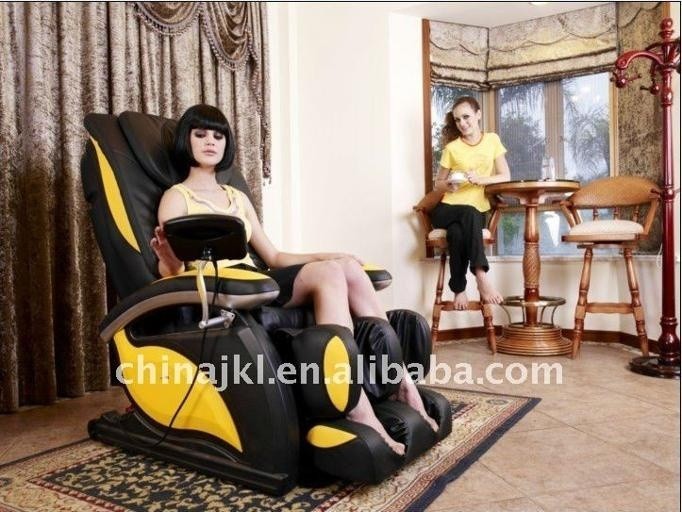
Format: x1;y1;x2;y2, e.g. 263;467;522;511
485;178;574;357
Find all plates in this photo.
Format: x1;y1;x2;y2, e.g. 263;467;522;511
449;180;467;183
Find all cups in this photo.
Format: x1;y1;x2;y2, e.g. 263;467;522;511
452;173;465;180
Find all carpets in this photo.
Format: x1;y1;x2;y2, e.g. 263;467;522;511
1;376;543;512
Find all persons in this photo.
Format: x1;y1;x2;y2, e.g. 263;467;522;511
150;105;440;455
430;96;511;310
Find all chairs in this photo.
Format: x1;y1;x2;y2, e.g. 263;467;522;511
415;183;502;354
561;175;662;362
81;111;452;497
